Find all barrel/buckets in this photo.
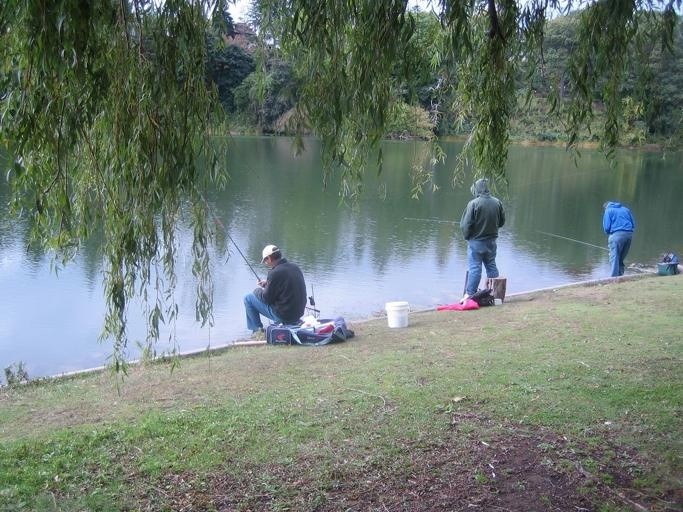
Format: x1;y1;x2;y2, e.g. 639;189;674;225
384;301;411;328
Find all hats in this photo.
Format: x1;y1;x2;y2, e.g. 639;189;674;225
260;244;280;264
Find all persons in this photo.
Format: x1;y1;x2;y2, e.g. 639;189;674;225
458;177;505;305
599;199;635;279
241;243;308;341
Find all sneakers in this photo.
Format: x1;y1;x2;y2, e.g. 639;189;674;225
248;329;265;340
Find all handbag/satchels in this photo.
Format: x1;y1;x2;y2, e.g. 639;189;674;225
459;289;494;306
264;324;292;347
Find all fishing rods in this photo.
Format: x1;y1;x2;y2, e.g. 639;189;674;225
403;216;608;253
189;184;264;288
